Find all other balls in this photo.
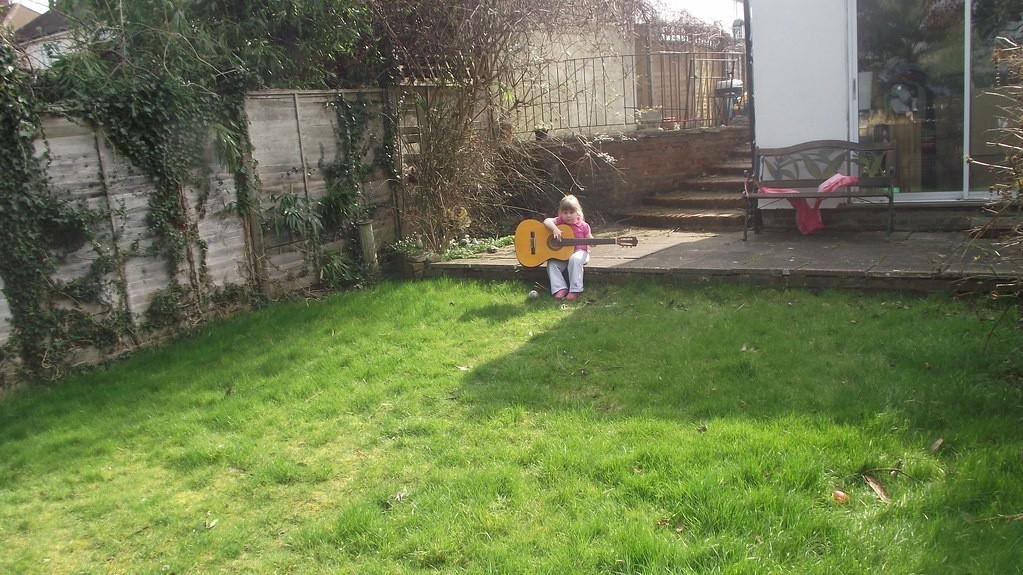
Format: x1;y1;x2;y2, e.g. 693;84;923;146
528;290;540;301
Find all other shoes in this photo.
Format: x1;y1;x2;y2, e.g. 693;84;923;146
555;289;569;298
566;293;579;300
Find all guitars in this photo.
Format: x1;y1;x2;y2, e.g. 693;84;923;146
513;218;638;269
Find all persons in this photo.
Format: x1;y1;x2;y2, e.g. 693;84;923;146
543;194;597;302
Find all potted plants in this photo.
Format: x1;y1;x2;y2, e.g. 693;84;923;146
635;105;665;133
535;120;549;140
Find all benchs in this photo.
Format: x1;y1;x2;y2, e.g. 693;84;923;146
742;138;898;241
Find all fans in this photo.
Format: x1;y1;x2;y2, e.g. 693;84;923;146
882;79;918;119
